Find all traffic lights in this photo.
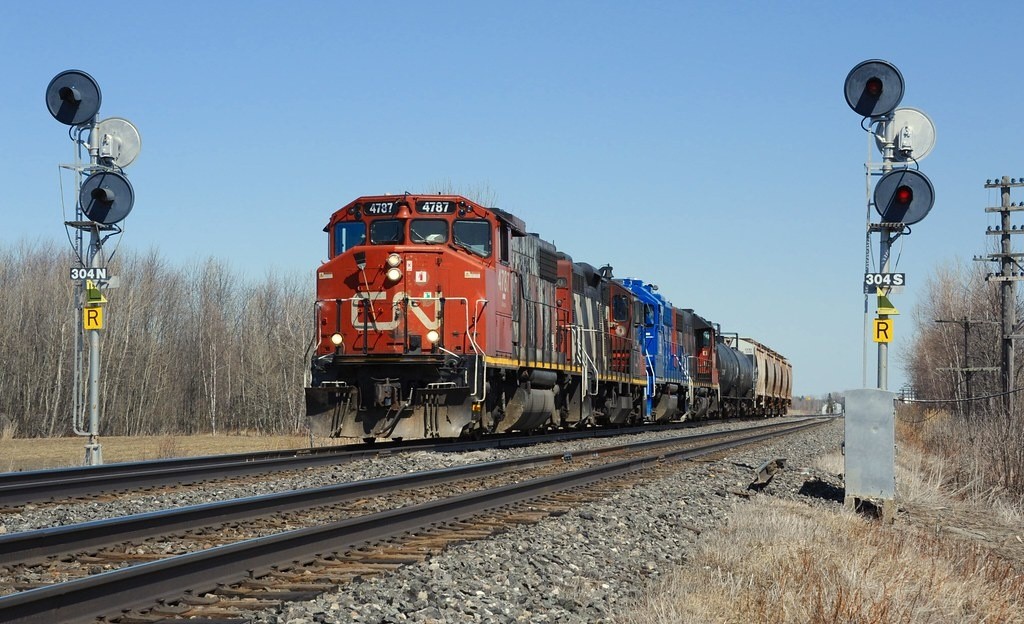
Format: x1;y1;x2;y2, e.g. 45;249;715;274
843;57;937;225
46;68;141;226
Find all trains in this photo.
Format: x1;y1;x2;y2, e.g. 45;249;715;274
303;191;792;443
